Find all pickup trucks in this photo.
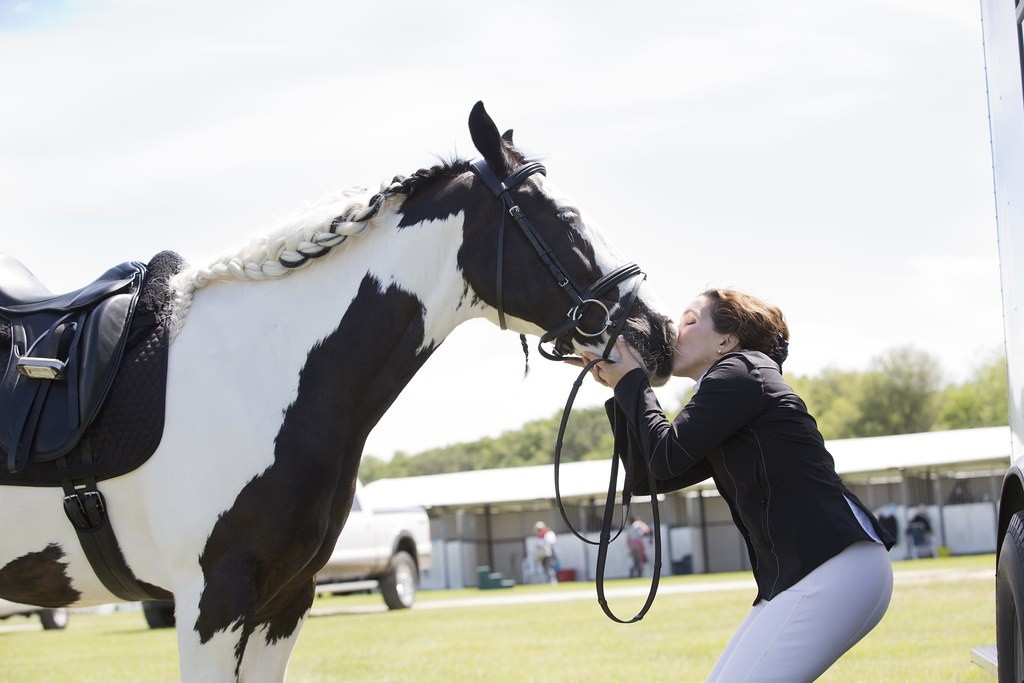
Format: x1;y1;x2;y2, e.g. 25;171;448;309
140;476;434;632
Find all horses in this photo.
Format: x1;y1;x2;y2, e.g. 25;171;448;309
0;100;680;682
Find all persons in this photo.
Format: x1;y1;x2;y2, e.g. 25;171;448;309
563;288;894;683
627;512;653;578
533;520;558;582
